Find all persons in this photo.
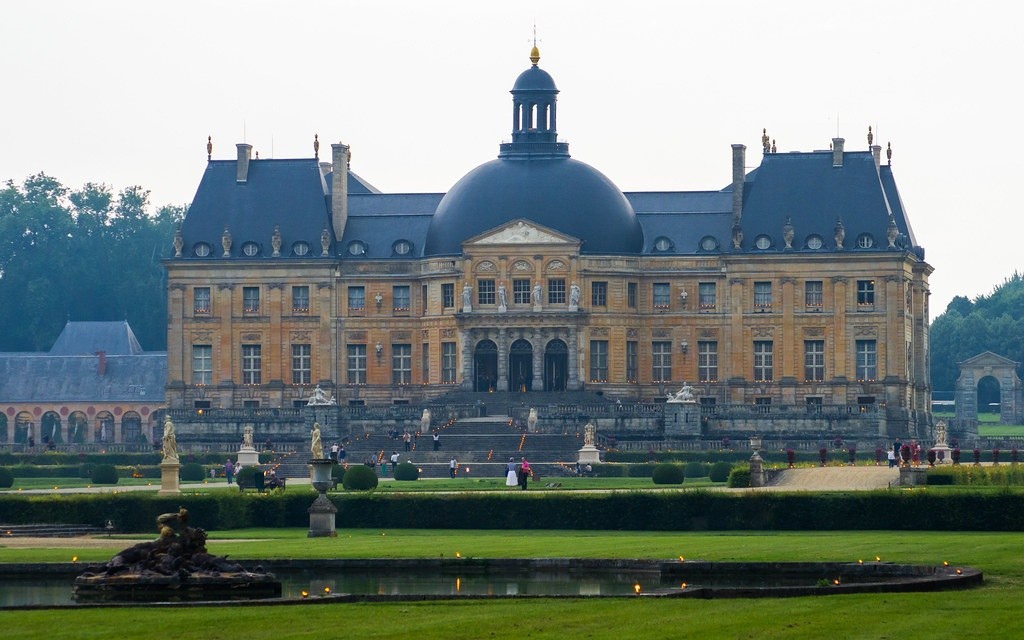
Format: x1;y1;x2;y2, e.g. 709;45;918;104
888;438;921;467
311;423;323;459
242;425;254;445
210;424;530;491
527;408;539;431
530;281;542;303
495;282;507;305
584;423;595;445
160;414;179;464
420;408;431;431
461;283;472;307
570;282;580;304
308;384;329;403
675;381;695;401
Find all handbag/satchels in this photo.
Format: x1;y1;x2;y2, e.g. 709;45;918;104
504;467;509;477
